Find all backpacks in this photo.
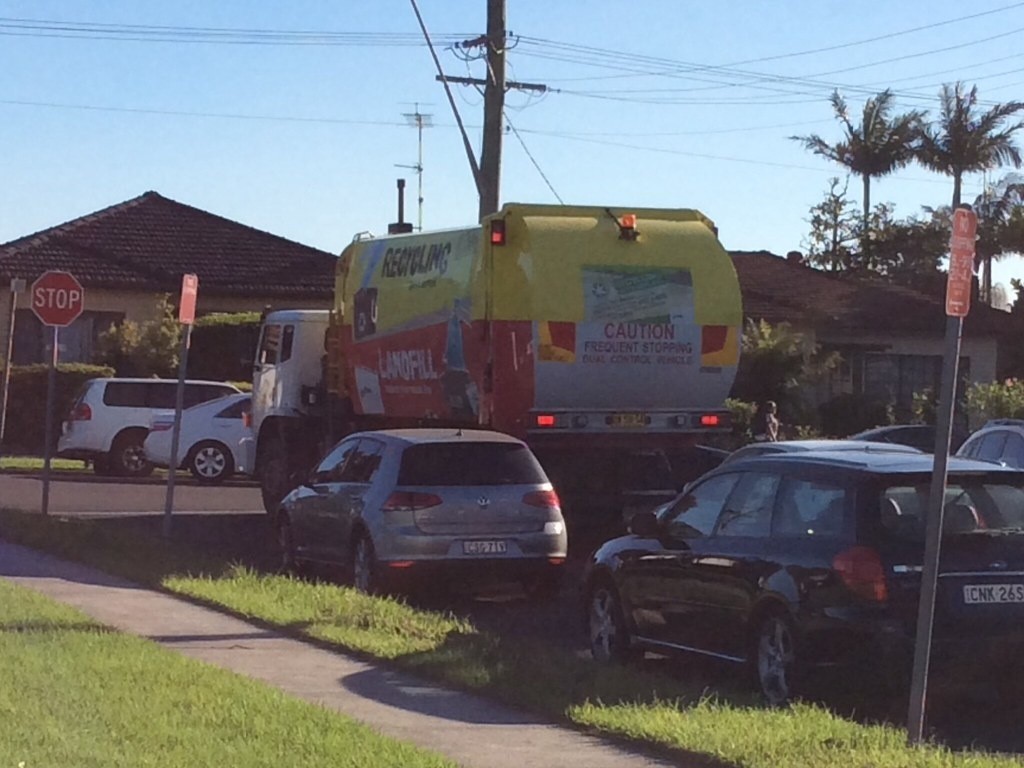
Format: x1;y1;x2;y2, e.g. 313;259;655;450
751;411;768;435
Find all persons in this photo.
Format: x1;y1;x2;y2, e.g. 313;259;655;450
754;401;778;442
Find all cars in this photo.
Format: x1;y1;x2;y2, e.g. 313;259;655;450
141;392;255;482
578;415;1024;543
273;427;567;609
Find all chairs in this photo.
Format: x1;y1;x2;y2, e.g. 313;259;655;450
943;504;979;534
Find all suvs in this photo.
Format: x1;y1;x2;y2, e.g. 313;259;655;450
580;449;1024;714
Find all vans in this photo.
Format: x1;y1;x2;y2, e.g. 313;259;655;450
55;378;247;479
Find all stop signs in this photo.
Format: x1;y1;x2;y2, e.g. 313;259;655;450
31;270;85;326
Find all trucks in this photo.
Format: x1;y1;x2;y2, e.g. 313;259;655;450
239;199;748;537
